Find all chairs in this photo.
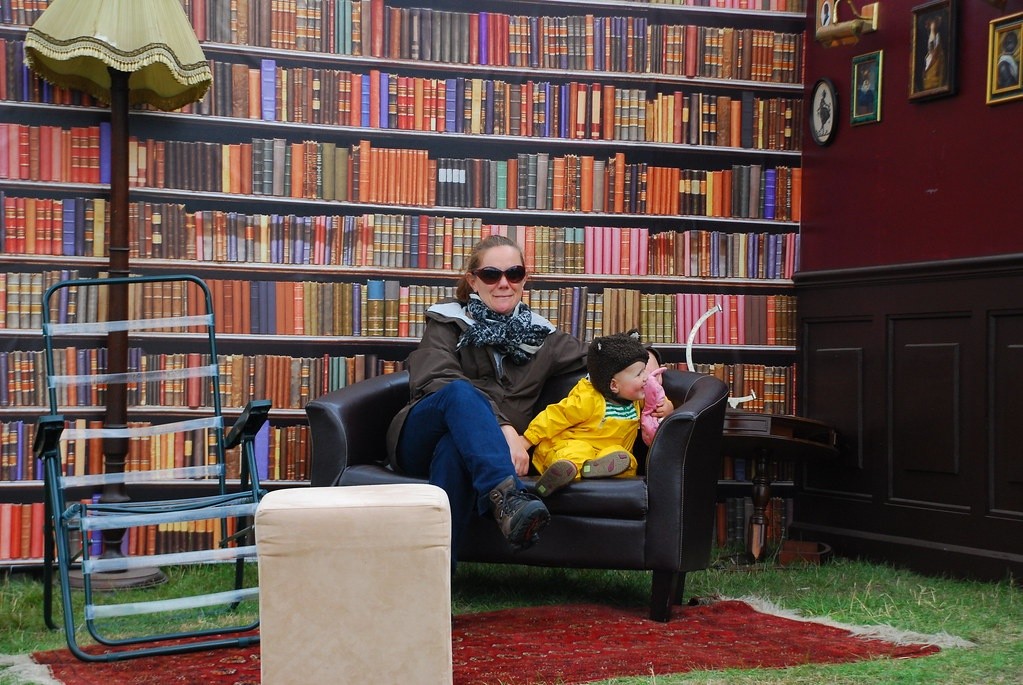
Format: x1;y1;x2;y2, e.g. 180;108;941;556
32;273;276;663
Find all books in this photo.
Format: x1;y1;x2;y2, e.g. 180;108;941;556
0;1;809;572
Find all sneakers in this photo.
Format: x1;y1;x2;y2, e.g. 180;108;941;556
489;475;551;554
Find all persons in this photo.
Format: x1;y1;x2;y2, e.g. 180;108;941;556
823;4;829;25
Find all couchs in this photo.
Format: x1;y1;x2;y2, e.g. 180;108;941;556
301;352;730;627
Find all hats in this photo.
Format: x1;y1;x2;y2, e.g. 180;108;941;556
588;328;649;405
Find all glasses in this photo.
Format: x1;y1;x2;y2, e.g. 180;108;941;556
471;265;526;285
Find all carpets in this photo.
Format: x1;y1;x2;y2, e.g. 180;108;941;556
0;601;979;685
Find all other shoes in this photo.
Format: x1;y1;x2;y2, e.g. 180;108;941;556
535;460;578;498
580;452;630;479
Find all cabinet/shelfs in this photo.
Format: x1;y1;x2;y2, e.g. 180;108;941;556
1;1;810;569
789;253;1022;587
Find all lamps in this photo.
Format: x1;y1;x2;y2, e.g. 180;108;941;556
22;0;216;594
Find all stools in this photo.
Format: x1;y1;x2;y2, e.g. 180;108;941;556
254;484;457;685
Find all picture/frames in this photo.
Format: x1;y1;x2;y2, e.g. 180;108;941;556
984;11;1023;105
906;0;957;104
808;75;839;148
848;50;884;128
815;0;837;42
